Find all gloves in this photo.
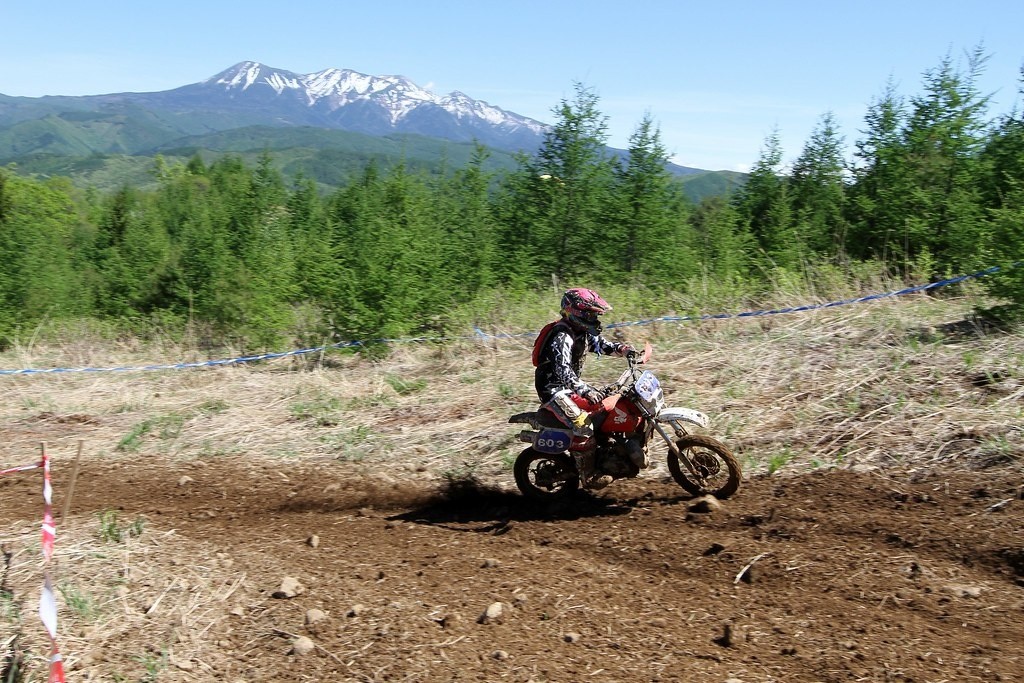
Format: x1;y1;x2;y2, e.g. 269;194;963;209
614;342;641;359
576;384;607;405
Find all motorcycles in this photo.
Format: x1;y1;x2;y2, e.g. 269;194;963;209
508;340;744;499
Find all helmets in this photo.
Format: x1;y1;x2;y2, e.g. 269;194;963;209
559;287;613;336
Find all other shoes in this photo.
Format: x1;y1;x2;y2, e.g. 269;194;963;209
582;472;613;490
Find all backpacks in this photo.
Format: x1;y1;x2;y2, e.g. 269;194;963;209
532;320;560;368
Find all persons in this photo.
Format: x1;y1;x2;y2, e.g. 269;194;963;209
535;288;636;490
